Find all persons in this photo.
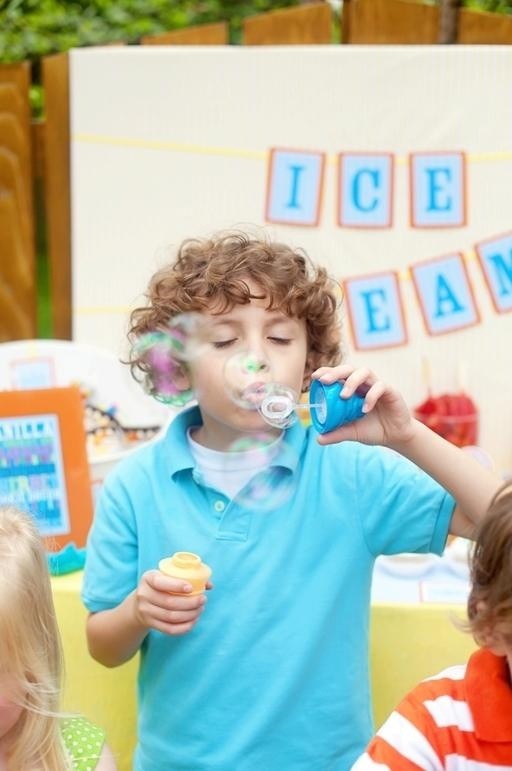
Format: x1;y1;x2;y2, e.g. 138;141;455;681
0;502;121;771
76;217;512;771
340;480;512;771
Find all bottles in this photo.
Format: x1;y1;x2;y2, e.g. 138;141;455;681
156;551;213;605
